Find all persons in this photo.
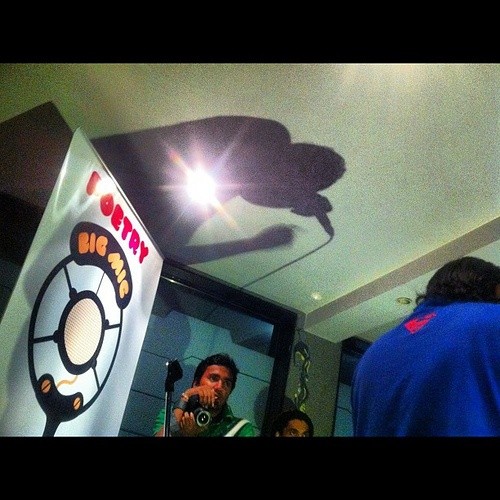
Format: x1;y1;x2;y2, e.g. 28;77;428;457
150;353;255;437
350;256;500;438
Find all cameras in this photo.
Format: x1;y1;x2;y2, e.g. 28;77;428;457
185;396;213;428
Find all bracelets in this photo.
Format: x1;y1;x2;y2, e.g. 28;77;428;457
171;399;191;413
179;392;191;401
268;409;314;438
